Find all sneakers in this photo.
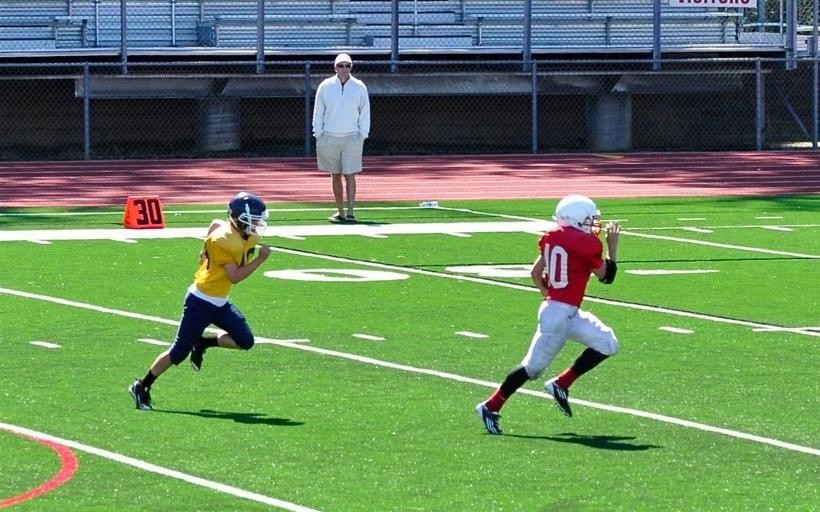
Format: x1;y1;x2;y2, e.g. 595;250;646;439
544;377;572;418
329;214;356;222
128;380;152;410
476;403;502;434
191;336;204;370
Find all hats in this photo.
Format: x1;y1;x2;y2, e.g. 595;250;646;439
334;54;353;72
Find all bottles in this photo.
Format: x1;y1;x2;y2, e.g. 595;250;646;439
419;201;437;208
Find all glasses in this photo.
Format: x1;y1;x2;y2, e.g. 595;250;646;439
337;64;350;68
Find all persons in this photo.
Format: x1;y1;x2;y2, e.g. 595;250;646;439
475;193;622;434
129;192;272;409
311;54;372;223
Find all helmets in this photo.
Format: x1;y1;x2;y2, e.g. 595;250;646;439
227;191;269;237
555;195;600;235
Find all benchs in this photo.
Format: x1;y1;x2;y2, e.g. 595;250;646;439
0;0;820;53
